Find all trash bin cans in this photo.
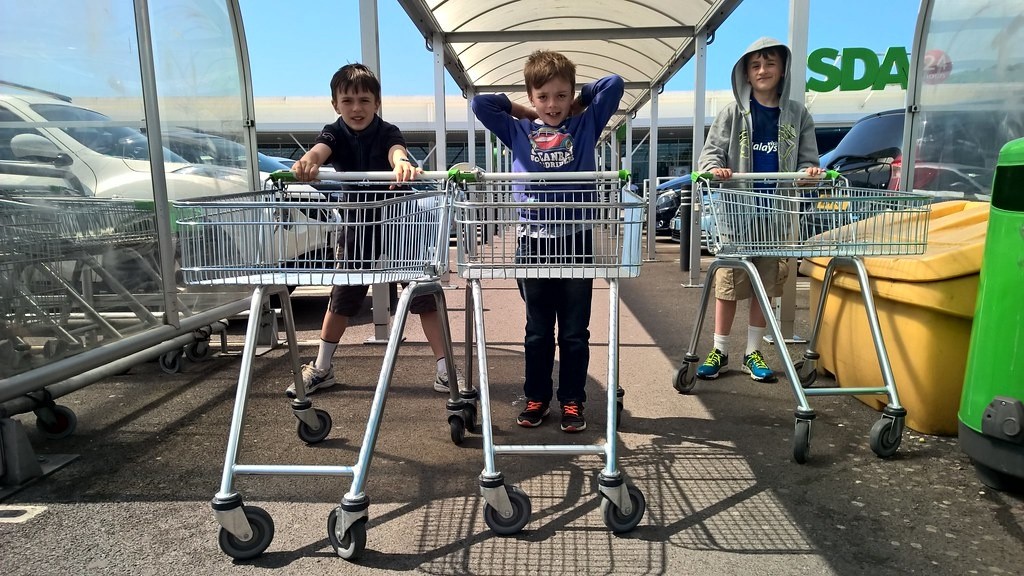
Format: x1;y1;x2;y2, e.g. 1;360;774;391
957;136;1024;492
799;193;995;439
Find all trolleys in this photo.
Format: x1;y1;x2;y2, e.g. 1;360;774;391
0;193;215;441
171;170;463;564
455;169;645;537
672;171;935;465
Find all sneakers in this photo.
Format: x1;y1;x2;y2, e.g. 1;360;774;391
286;361;335;398
434;365;478;395
517;400;550;427
740;350;775;381
696;347;729;378
560;399;586;431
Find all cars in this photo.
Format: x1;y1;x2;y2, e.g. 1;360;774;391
158;128;438;222
669;149;833;256
0;92;343;318
801;100;1024;245
655;172;691;237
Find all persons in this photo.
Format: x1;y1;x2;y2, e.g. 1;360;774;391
472;51;624;429
285;63;479;398
693;36;830;379
